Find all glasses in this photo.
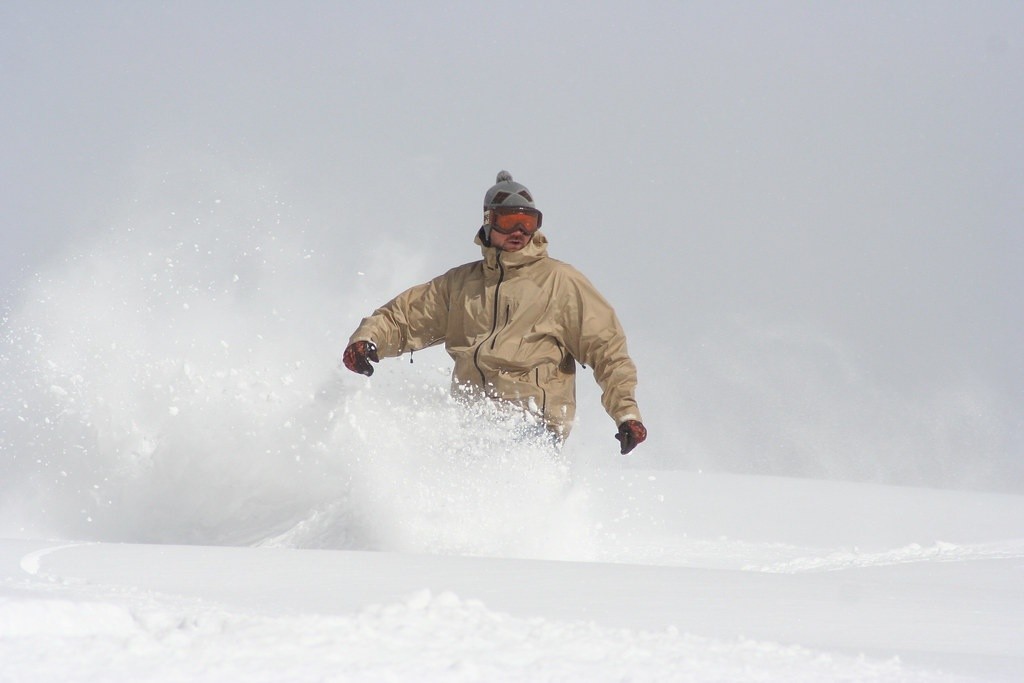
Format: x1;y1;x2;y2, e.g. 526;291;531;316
484;206;543;235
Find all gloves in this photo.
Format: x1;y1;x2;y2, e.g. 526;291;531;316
342;340;379;378
615;420;648;455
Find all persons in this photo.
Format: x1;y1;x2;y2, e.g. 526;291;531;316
341;171;648;456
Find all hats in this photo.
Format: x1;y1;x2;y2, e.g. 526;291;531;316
483;171;535;241
